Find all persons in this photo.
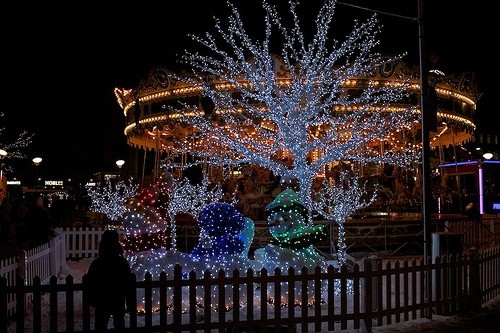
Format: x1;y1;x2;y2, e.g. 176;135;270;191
0;193;77;247
87;229;137;333
